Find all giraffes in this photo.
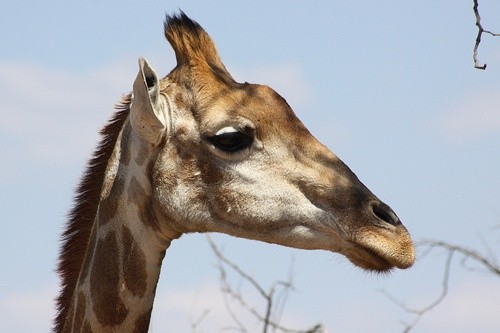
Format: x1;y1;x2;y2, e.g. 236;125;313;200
51;7;418;332
